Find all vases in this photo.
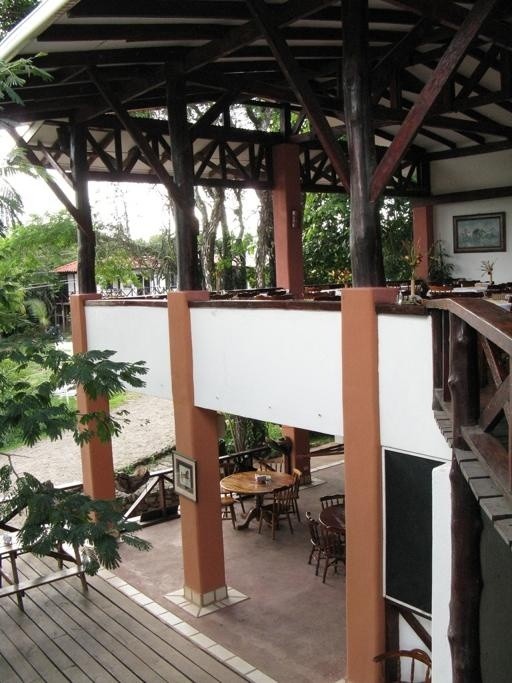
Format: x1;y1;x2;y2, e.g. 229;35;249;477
488;274;492;283
410;277;415;296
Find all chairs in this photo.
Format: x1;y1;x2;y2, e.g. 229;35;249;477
259;458;283;472
272;468;302;523
259;483;294;541
217;460;246;517
206;275;458;310
372;645;432;683
318;523;346;583
304;511;340;576
319;493;345;511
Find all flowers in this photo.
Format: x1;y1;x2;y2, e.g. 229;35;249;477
479;258;495;277
332;267;352;288
400;239;424;279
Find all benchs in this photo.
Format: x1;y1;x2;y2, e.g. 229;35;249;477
1;564;89;612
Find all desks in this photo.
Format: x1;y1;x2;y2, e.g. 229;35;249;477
0;522;82;586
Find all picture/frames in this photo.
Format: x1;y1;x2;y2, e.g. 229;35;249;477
452;211;506;252
171;449;198;503
